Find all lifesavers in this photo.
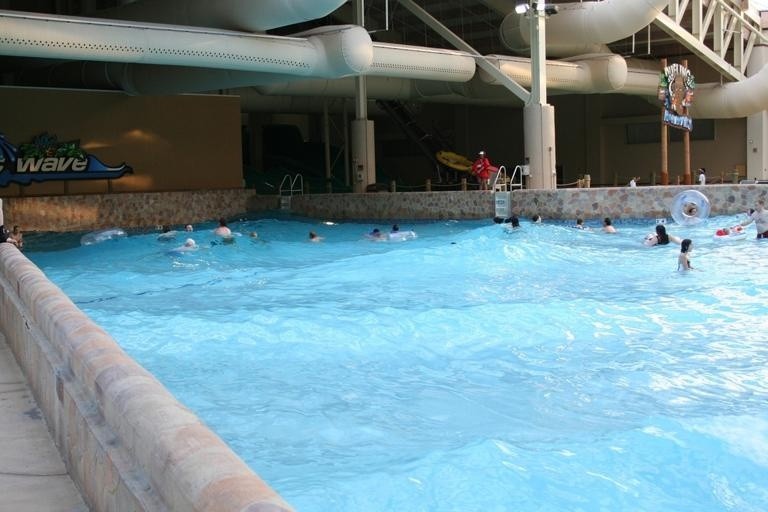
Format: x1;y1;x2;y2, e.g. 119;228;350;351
713;230;747;240
81;228;128;247
670;190;710;225
387;230;415;239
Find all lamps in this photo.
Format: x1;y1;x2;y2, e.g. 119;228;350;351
514;2;561;20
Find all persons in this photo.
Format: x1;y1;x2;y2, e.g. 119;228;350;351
157;222;181;239
249;232;261;241
0;223;17;244
367;228;386;242
184;223;194;232
10;225;25;249
472;150;494;190
212;218;234;245
389;224;406;241
167;237;199;252
493;166;768;246
308;230;326;243
676;238;693;269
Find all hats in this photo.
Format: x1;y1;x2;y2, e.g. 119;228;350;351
698;169;705;173
478;151;484;155
